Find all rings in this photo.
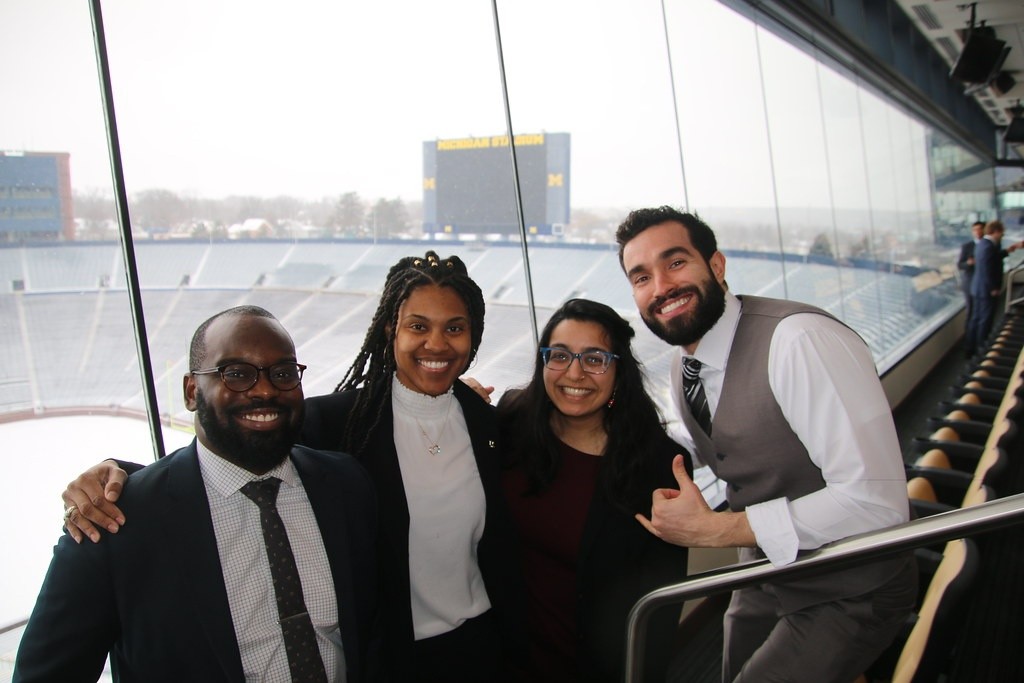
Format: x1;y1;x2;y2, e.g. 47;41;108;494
65;506;78;517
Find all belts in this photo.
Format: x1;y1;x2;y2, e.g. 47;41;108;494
414;608;497;642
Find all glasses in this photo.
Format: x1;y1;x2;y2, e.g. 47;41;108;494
539;347;620;375
191;360;306;393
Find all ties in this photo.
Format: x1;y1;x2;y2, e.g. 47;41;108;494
240;476;327;683
681;356;713;438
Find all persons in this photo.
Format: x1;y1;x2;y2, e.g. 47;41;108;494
61;252;510;683
13;305;392;683
460;298;693;681
956;221;1024;360
498;206;917;683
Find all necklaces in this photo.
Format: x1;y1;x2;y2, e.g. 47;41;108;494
393;392;452;455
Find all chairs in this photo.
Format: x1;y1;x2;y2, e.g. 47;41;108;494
855;311;1024;683
